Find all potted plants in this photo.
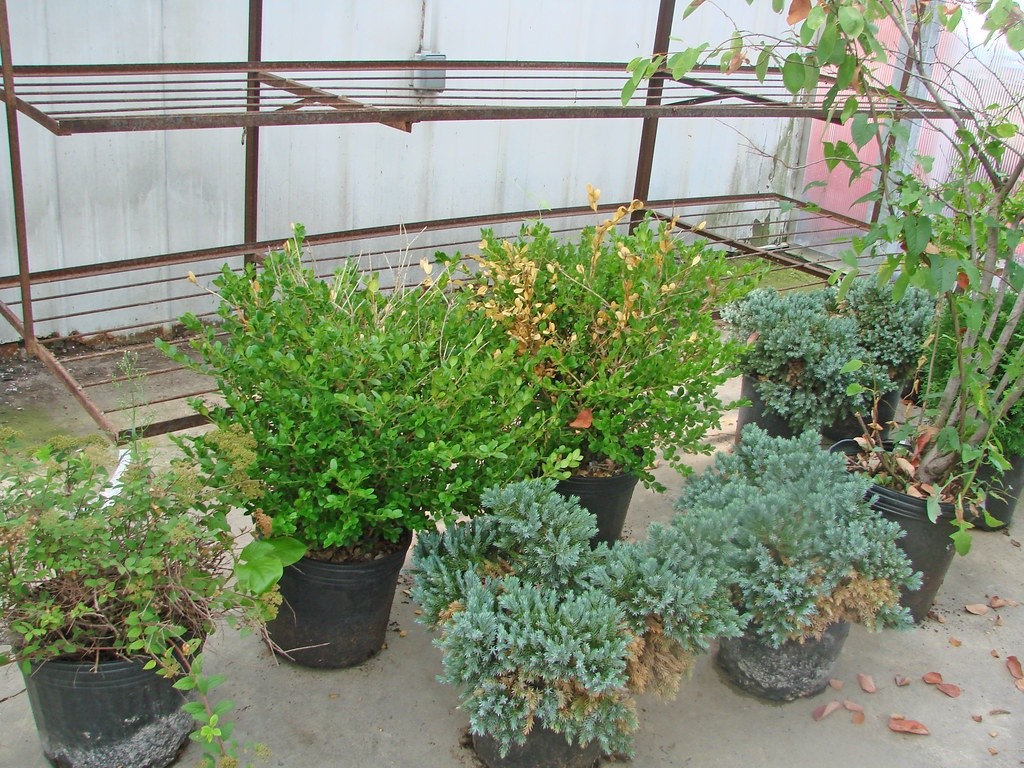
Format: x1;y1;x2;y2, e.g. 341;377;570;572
0;1;1024;768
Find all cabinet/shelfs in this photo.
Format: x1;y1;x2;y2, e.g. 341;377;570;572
0;1;976;434
